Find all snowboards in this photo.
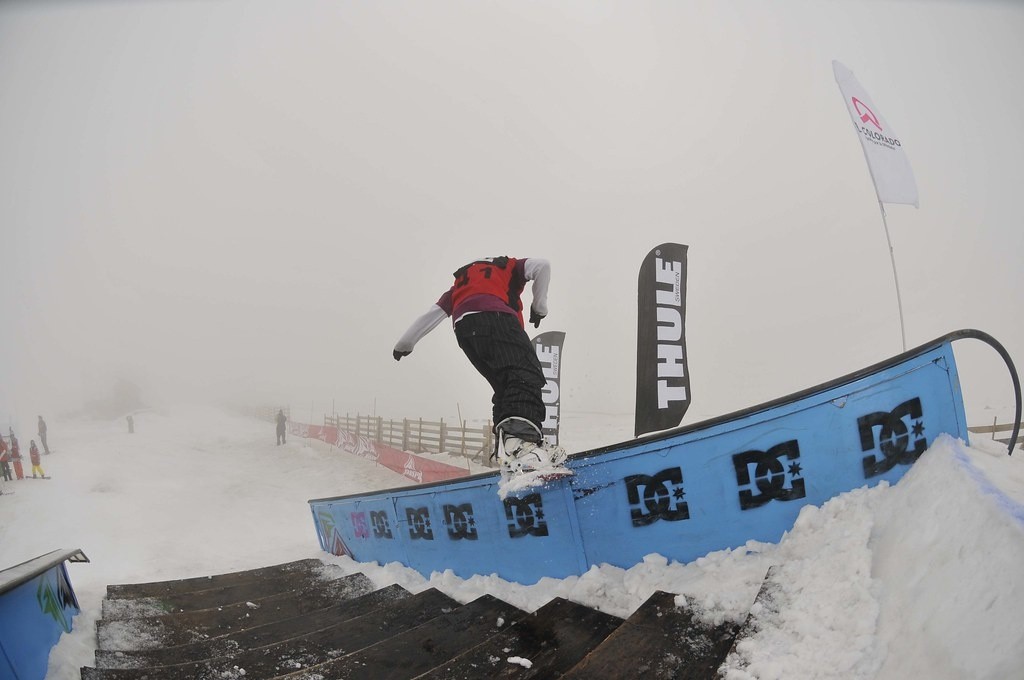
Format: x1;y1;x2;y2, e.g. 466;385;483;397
498;465;576;480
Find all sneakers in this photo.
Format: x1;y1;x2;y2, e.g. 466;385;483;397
499;435;567;473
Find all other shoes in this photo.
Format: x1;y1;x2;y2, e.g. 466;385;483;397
33;474;37;478
41;475;45;479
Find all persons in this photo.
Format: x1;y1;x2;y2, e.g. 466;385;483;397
391;255;552;428
274;410;286;445
0;415;50;479
126;416;134;433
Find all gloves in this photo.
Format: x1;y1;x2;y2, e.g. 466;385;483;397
529;305;546;328
393;349;412;361
